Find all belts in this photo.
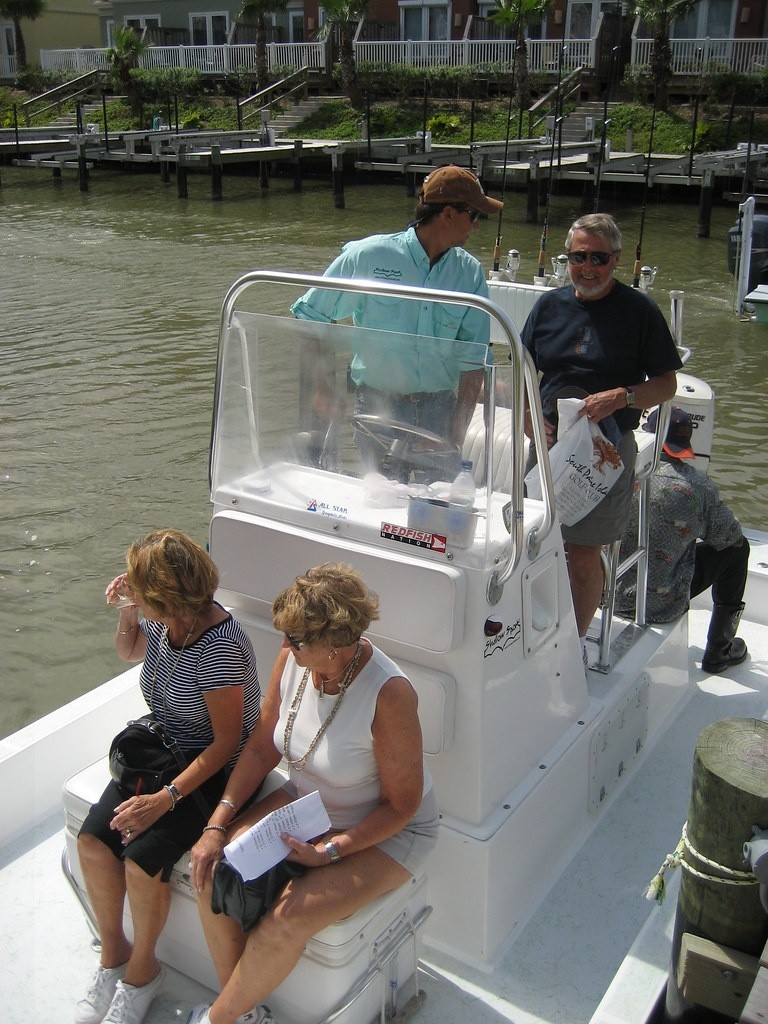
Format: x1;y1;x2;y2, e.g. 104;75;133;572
358;382;456;404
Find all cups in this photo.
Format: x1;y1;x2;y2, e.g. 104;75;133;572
108;576;138;609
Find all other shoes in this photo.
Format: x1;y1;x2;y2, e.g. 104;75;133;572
582;645;589;678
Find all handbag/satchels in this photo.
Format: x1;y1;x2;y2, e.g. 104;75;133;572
109;712;201;796
523;398;625;527
211;857;305;933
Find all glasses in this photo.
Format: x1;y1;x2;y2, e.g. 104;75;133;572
285;632;308;651
464;209;481;224
567;250;616;266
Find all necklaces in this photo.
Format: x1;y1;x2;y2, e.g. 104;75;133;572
282;640;364;773
150;614;199;731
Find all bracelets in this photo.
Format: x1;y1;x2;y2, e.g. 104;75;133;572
118;620;140;635
324;840;341;863
202;826;227;835
219;799;237;813
164;783;183;810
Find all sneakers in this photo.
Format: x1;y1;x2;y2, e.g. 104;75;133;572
186;998;213;1024
100;968;164;1024
84;962;128;1024
254;1004;277;1024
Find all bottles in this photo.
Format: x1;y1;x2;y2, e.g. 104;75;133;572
448;460;476;512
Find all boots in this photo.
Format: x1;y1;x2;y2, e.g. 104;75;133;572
702;601;748;673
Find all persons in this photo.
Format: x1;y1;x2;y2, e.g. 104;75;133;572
182;559;441;1024
290;164;505;487
74;528;266;1024
613;405;750;674
520;211;684;685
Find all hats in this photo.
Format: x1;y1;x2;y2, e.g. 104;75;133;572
641;406;696;458
419;164;504;215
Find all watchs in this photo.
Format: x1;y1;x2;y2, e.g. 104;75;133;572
622;385;637;408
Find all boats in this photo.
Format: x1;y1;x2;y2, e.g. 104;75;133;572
726;215;768;323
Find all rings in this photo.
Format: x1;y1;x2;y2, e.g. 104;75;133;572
587;413;592;418
125;827;130;834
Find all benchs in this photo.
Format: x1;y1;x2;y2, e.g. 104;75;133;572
62;754;428;1024
461;278;692;497
